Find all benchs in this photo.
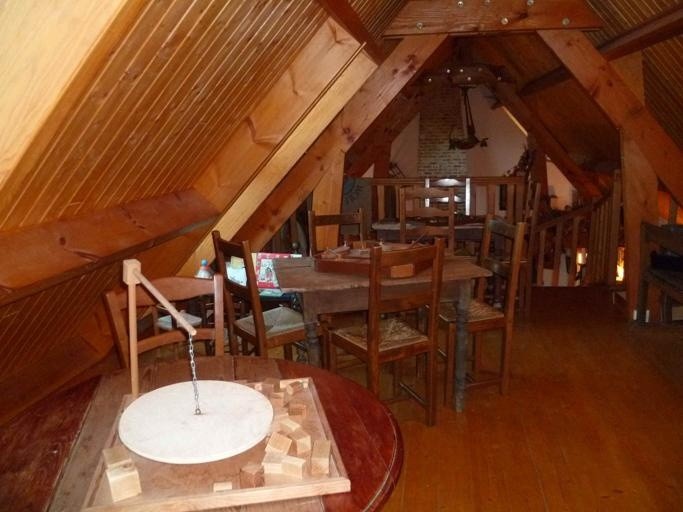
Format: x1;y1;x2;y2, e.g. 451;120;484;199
637;220;683;328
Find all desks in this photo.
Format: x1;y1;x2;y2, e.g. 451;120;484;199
2;356;404;512
224;238;494;414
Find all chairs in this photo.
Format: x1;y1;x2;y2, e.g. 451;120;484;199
399;187;454;253
326;235;446;427
308;207;369;256
478;180;542;312
424;177;471;216
416;212;527;409
212;230;329;370
101;273;225;368
